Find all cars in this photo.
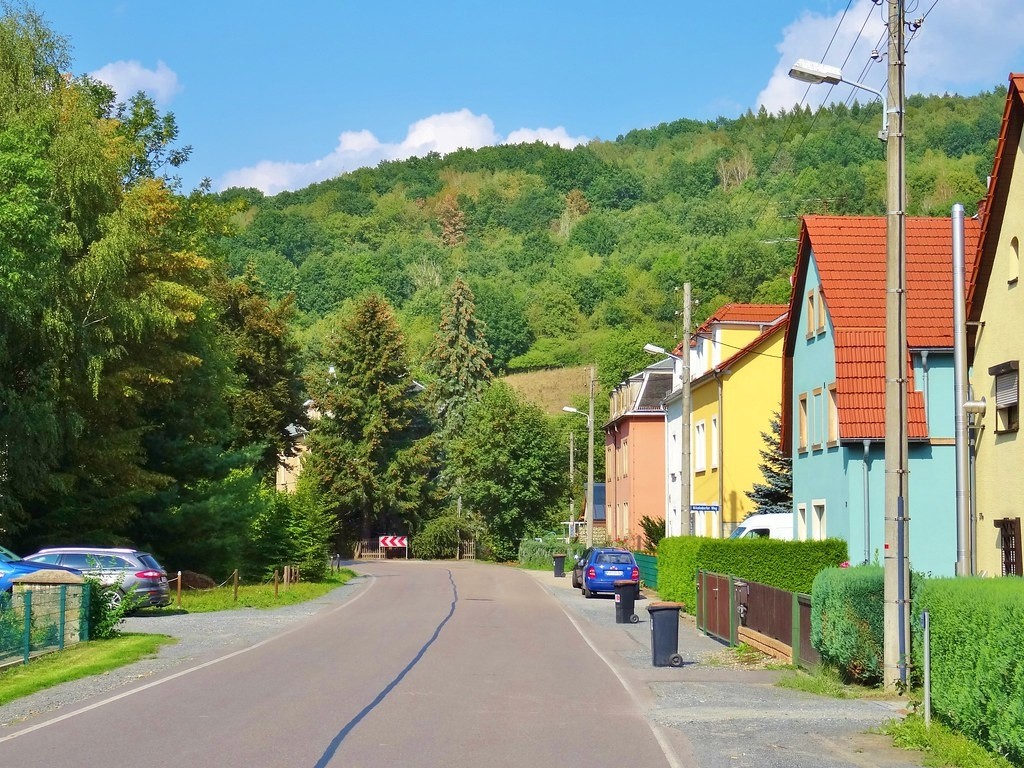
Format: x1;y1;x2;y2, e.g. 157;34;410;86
581;549;640;599
24;547;173;618
572;547;594;589
0;545;81;593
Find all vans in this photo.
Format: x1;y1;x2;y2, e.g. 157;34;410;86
726;513;793;543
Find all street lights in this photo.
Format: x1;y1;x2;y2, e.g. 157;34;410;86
788;58;910;697
642;345;692;535
562;406;593;547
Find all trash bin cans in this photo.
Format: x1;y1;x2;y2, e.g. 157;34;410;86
612;579;640;624
647;601;684;667
552;554;566;578
